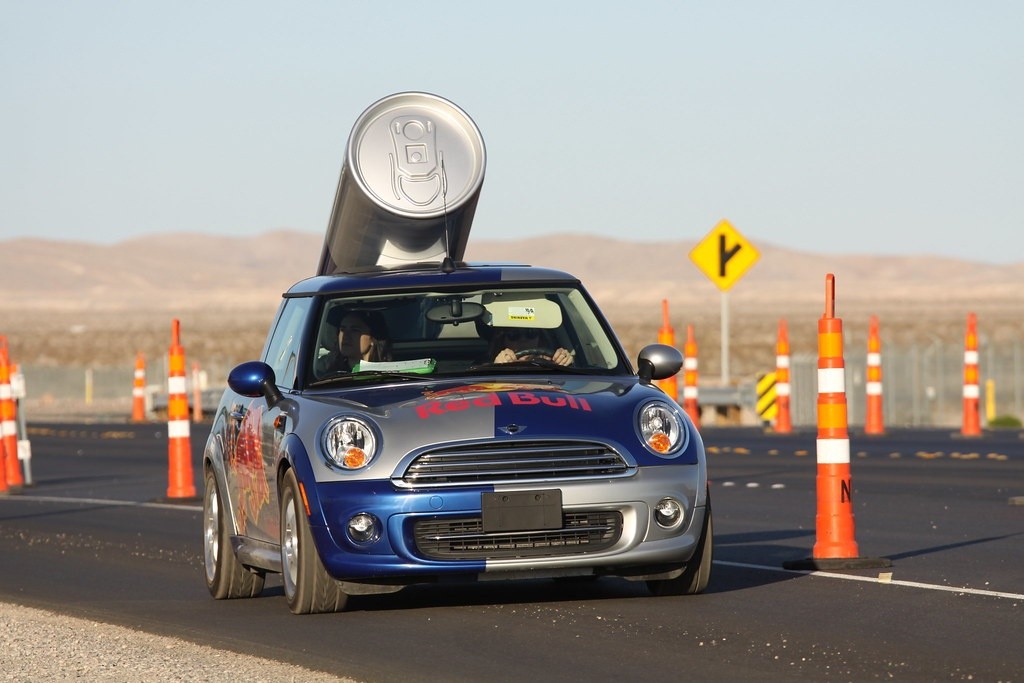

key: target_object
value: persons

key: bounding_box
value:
[319,309,392,375]
[493,327,575,367]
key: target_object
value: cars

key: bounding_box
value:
[202,260,711,616]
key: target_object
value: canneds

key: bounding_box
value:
[317,90,487,352]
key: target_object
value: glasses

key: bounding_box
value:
[506,329,539,341]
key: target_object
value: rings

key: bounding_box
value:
[562,353,567,356]
[505,353,509,356]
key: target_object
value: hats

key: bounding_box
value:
[326,302,388,340]
